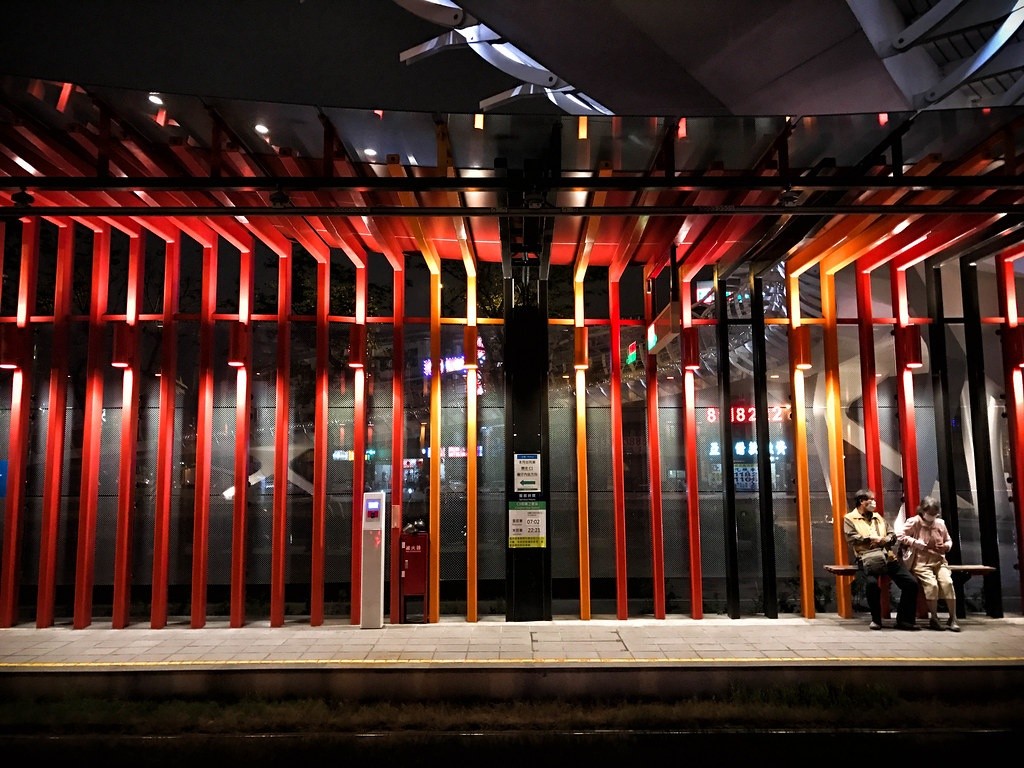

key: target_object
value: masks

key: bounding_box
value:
[923,512,940,522]
[863,499,876,512]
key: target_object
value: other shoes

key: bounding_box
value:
[945,618,961,632]
[893,620,921,630]
[868,621,882,629]
[928,618,946,631]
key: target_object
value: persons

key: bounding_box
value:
[843,489,921,630]
[896,495,960,632]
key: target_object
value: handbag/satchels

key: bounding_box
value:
[893,517,919,570]
[860,548,887,575]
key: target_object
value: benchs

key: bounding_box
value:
[823,564,995,619]
